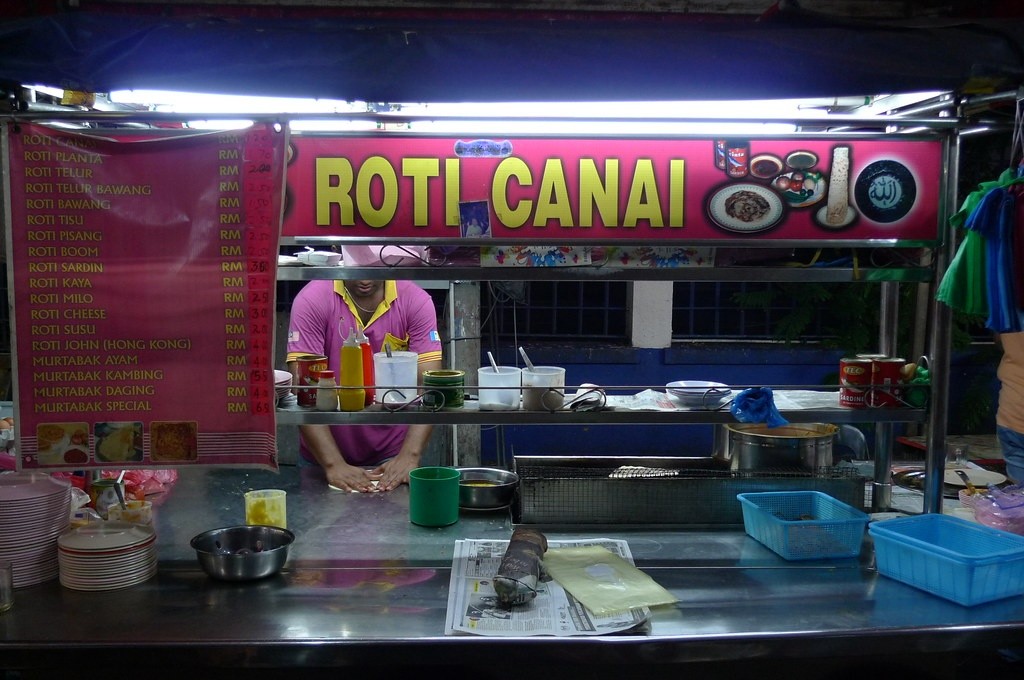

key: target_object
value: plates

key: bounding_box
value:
[0,472,157,589]
[785,150,819,170]
[771,171,828,208]
[274,369,294,402]
[703,180,786,234]
[749,153,783,179]
[666,380,729,405]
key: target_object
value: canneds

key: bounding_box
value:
[92,478,125,520]
[838,352,906,408]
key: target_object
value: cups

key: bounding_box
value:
[409,467,460,528]
[0,561,15,614]
[244,489,287,531]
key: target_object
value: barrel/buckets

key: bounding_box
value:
[107,501,154,528]
[91,479,125,521]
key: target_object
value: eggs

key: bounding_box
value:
[0,417,14,432]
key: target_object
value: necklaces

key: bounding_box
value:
[344,286,375,312]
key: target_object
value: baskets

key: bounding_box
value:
[736,491,869,561]
[869,512,1024,607]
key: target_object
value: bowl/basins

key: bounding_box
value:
[451,467,520,507]
[191,525,295,583]
[725,424,839,470]
[521,366,567,410]
[373,351,420,405]
[478,366,522,412]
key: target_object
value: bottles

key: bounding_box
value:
[338,328,366,412]
[316,370,338,411]
[714,139,750,179]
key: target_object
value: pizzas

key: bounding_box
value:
[37,424,66,443]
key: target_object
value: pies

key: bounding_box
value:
[98,425,134,462]
[154,422,191,458]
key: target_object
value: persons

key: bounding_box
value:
[995,331,1024,483]
[293,466,436,595]
[287,277,442,493]
[466,217,482,237]
